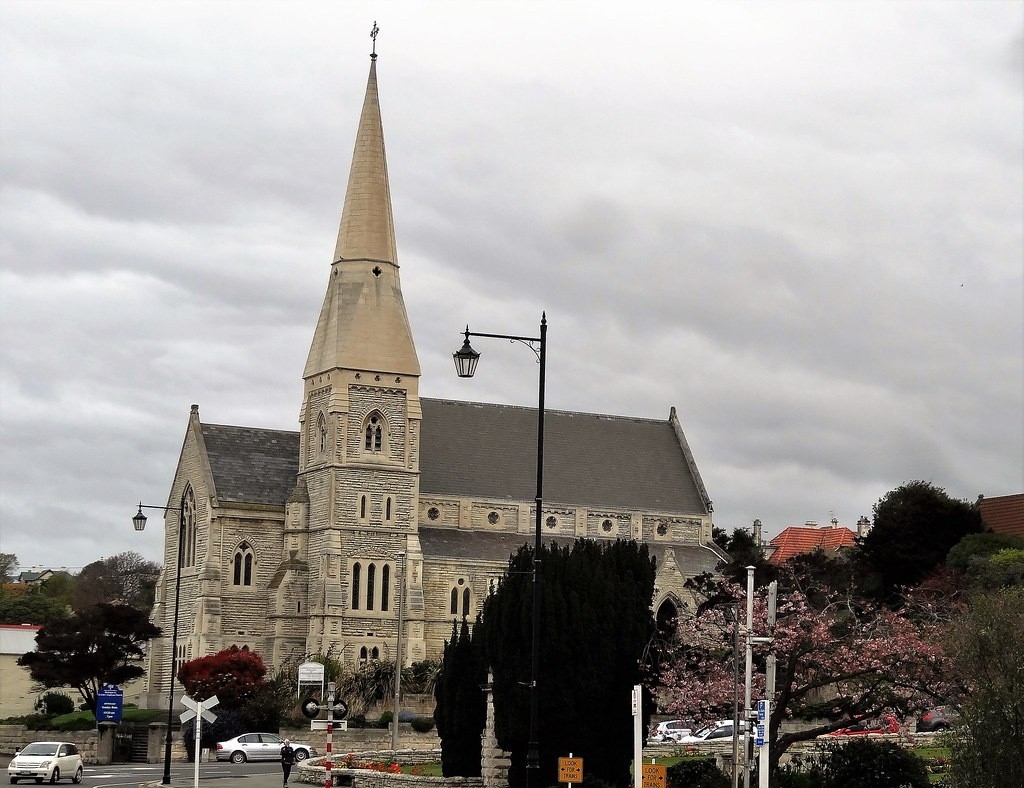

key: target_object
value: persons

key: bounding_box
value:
[280,738,295,788]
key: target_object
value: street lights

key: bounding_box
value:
[457,312,558,781]
[133,496,186,785]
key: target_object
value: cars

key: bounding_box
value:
[688,720,753,747]
[827,716,900,735]
[916,702,969,732]
[657,719,704,745]
[10,740,83,783]
[218,730,312,771]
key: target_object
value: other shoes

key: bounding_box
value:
[282,783,289,788]
[281,779,284,783]
